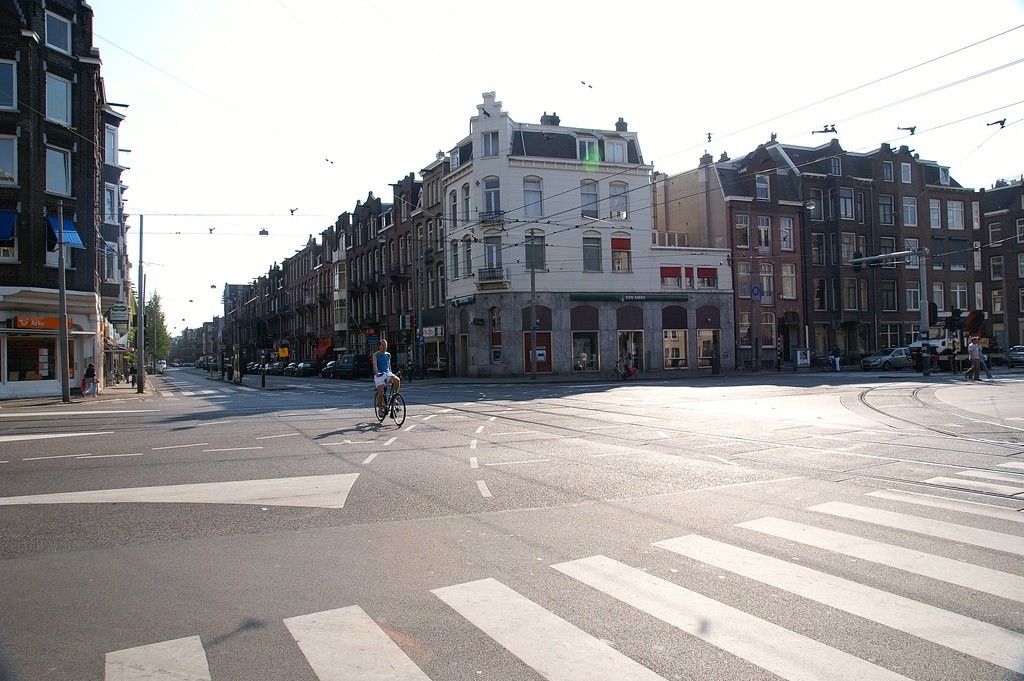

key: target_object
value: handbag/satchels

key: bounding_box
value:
[93,377,100,383]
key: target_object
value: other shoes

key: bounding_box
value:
[963,372,967,378]
[974,378,982,381]
[969,376,973,380]
[378,411,385,417]
[988,376,994,379]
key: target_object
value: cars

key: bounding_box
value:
[195,354,373,381]
[860,345,914,372]
[156,359,180,373]
[1004,345,1024,368]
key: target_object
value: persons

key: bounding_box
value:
[580,353,587,370]
[123,362,131,383]
[620,352,632,377]
[130,365,136,388]
[372,339,402,418]
[82,363,98,399]
[573,342,577,366]
[963,336,994,380]
[591,353,597,369]
[800,352,807,364]
[828,344,841,371]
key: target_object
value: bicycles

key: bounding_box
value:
[606,359,637,382]
[822,356,844,373]
[372,371,407,427]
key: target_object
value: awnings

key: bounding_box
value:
[103,337,129,353]
[313,345,332,354]
